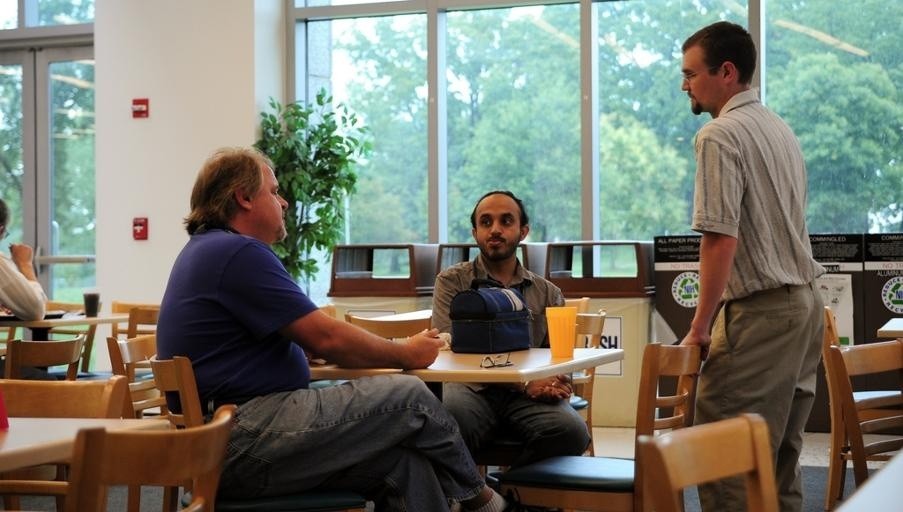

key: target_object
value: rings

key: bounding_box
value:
[550,381,557,387]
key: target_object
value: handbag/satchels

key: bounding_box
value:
[449,278,532,354]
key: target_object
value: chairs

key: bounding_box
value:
[636,410,780,511]
[822,306,902,512]
[563,296,590,400]
[568,308,607,457]
[344,313,432,341]
[499,343,702,511]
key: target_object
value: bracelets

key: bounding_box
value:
[524,380,531,394]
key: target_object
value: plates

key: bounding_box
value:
[0,310,66,320]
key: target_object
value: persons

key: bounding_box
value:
[157,145,563,512]
[430,190,588,510]
[678,20,826,512]
[0,199,60,382]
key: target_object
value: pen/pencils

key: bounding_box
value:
[527,309,534,320]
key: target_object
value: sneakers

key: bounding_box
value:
[501,493,564,511]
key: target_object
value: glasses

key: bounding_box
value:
[2,224,11,242]
[479,352,514,369]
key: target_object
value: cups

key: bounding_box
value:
[82,288,100,317]
[546,307,578,363]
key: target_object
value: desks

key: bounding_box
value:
[308,347,624,403]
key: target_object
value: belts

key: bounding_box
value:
[173,398,250,431]
